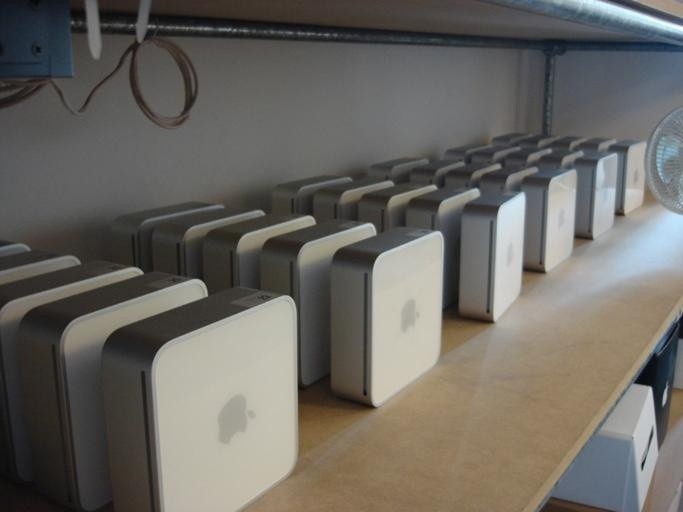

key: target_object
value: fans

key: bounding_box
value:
[644,106,683,215]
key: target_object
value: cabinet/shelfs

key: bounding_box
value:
[0,0,682,512]
